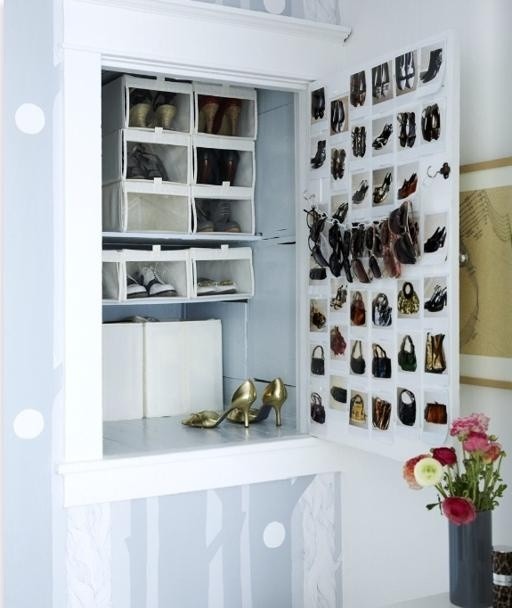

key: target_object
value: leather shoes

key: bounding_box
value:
[311,48,443,133]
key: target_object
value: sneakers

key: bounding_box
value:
[197,278,237,295]
[127,273,147,298]
[136,265,176,297]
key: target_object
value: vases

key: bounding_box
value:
[449,509,493,607]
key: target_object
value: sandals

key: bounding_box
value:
[129,89,153,127]
[331,148,346,181]
[351,103,440,158]
[153,91,178,130]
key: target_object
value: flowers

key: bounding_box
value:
[402,412,508,526]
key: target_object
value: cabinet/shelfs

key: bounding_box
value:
[55,0,462,506]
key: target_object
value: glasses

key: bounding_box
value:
[303,199,418,284]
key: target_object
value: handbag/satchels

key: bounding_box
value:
[123,144,169,181]
[350,291,366,325]
[311,300,326,329]
[310,267,327,280]
[372,293,392,326]
[397,282,419,314]
[309,326,447,430]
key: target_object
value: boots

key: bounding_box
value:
[311,140,327,170]
[192,199,214,232]
[198,149,219,183]
[210,200,240,232]
[217,150,240,185]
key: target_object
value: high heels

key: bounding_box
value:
[332,172,418,225]
[424,226,446,252]
[213,99,241,136]
[226,377,288,427]
[182,379,257,428]
[199,96,221,134]
[334,285,348,310]
[424,285,441,308]
[428,287,448,312]
[330,285,344,307]
